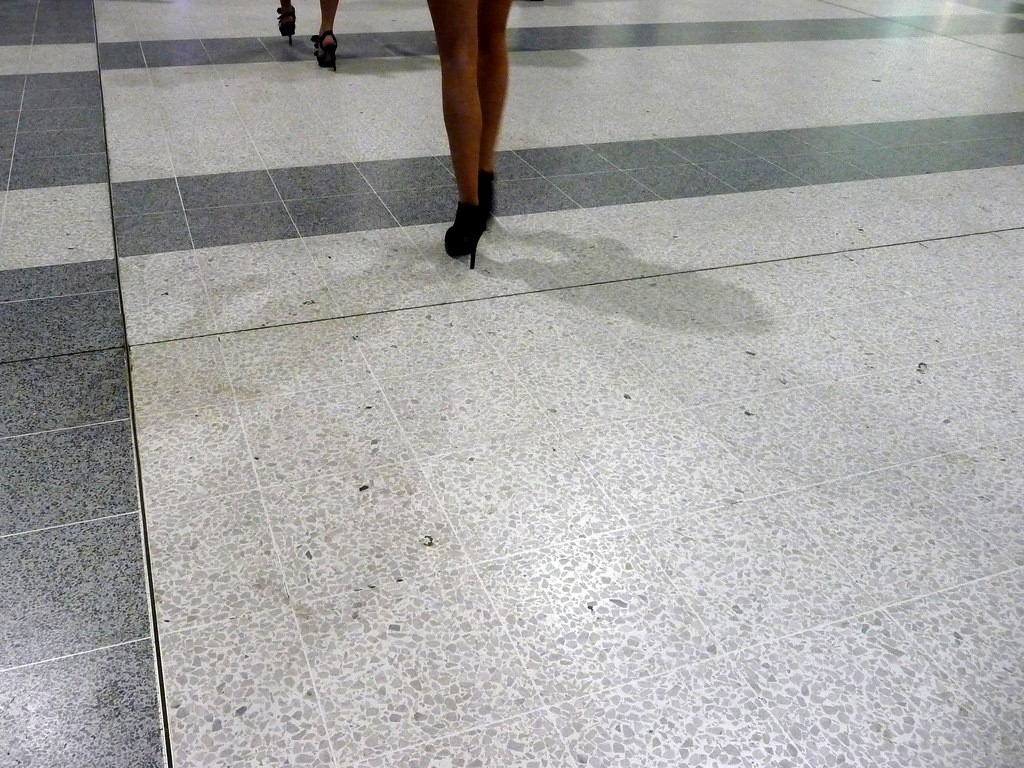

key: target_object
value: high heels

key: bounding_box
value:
[277,6,296,47]
[310,31,338,71]
[445,201,486,269]
[478,171,497,222]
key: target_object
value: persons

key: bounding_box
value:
[426,0,513,269]
[277,0,339,71]
